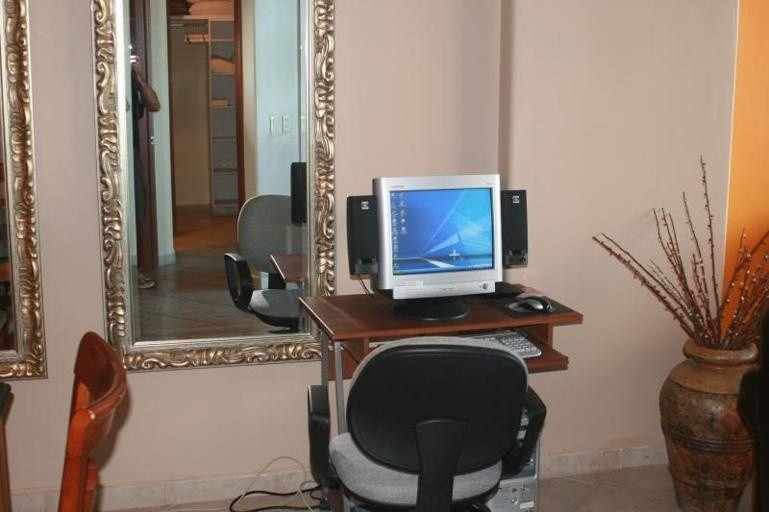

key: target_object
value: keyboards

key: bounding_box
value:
[377,330,541,359]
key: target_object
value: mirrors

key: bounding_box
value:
[0,0,49,381]
[88,0,338,374]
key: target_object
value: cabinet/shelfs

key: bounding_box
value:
[208,19,242,215]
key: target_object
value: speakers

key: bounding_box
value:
[501,190,528,269]
[347,196,379,274]
[291,162,306,225]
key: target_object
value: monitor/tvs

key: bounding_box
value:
[373,173,504,322]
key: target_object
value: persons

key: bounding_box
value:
[127,42,159,294]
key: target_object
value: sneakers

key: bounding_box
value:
[137,270,156,289]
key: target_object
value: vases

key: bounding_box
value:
[659,337,762,511]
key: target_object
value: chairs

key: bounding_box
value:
[56,330,128,510]
[305,335,548,510]
[222,195,304,333]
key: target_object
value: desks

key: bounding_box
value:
[295,283,583,507]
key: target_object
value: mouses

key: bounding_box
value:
[519,296,550,312]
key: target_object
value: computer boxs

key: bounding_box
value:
[483,407,540,512]
